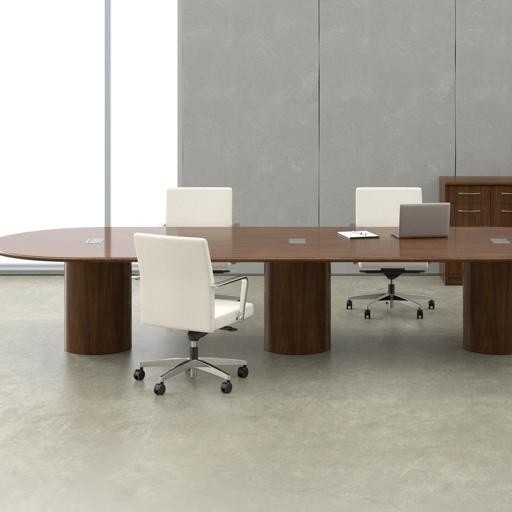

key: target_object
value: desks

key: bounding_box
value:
[0,226,512,355]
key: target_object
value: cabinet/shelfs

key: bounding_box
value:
[438,177,512,286]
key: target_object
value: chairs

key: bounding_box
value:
[132,233,253,396]
[346,188,435,320]
[164,186,233,335]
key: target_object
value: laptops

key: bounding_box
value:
[390,201,451,240]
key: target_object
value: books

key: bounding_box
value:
[336,230,378,239]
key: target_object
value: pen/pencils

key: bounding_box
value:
[360,231,366,236]
